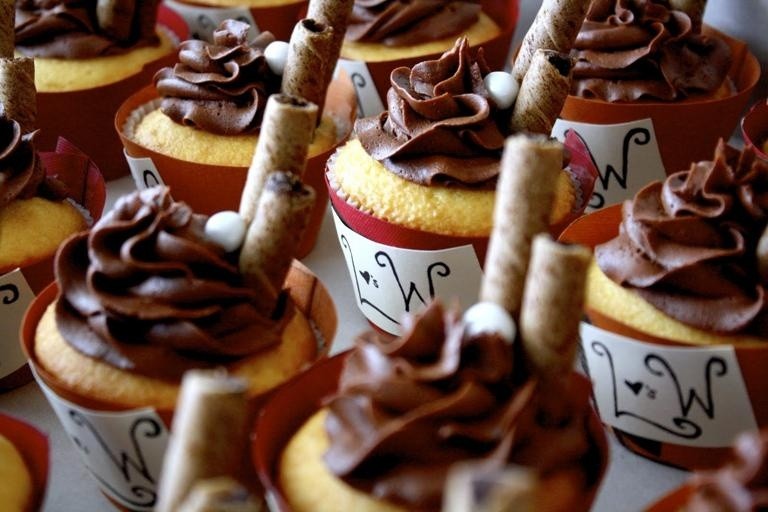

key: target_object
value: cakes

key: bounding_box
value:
[0,0,768,512]
